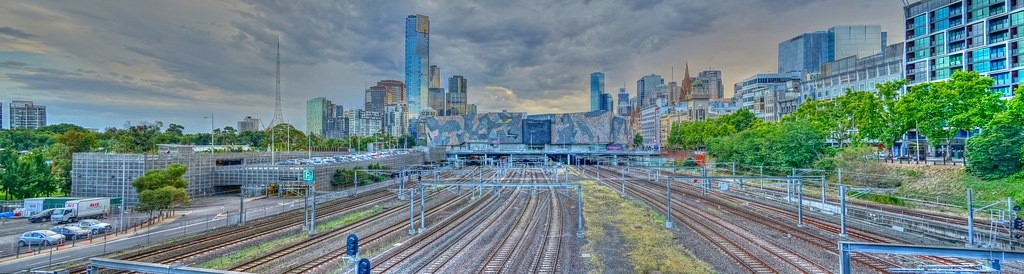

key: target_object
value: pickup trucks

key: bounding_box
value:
[66,219,112,234]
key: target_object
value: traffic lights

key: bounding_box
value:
[359,259,370,274]
[347,235,358,256]
[1014,218,1024,230]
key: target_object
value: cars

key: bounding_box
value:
[897,154,923,162]
[50,225,90,241]
[274,151,413,165]
[18,230,65,247]
[28,209,61,222]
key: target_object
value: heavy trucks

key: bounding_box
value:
[50,196,110,224]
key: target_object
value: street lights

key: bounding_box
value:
[203,114,214,155]
